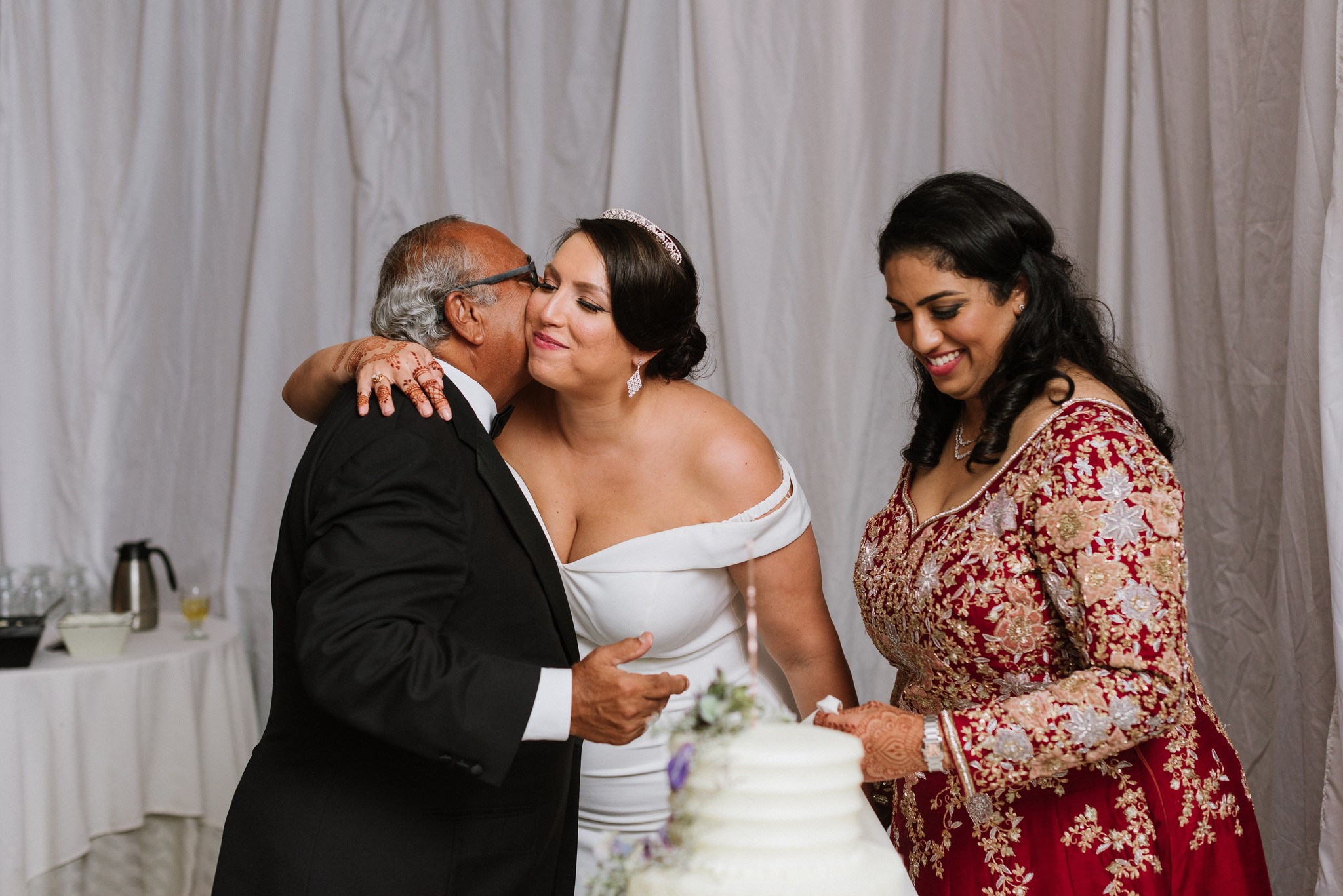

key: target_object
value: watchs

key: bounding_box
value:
[919,710,949,774]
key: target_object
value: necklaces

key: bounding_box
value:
[951,422,979,459]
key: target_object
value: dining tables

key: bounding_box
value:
[2,610,261,896]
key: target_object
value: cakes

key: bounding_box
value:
[596,720,916,896]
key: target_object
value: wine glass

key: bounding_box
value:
[178,583,211,642]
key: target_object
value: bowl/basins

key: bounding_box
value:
[58,607,133,662]
[1,613,45,668]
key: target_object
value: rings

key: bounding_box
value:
[369,372,393,389]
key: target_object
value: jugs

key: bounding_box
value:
[110,539,178,632]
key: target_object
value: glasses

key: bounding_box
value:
[428,253,544,322]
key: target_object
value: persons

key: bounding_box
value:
[811,171,1270,896]
[280,207,870,896]
[208,211,688,896]
